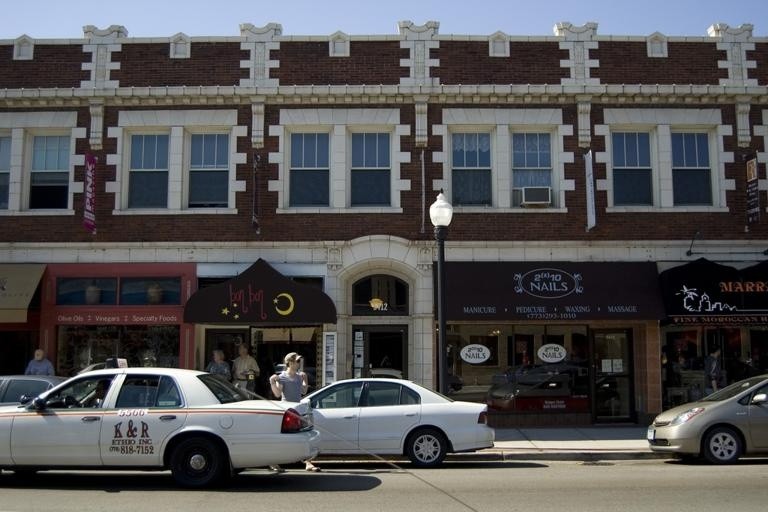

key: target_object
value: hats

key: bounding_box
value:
[285,352,302,363]
[35,349,43,360]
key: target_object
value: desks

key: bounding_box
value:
[680,371,705,400]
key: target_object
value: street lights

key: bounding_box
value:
[428,188,453,396]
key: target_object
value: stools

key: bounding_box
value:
[667,387,689,408]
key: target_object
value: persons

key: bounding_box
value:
[232,343,259,392]
[724,350,768,385]
[703,345,723,398]
[23,349,55,376]
[67,377,110,409]
[267,351,322,473]
[205,348,232,385]
[521,349,531,366]
[380,355,393,378]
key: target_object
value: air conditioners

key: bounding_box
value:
[520,186,552,205]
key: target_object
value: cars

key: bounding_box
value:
[0,357,320,489]
[76,362,106,374]
[369,369,402,379]
[646,374,767,465]
[486,365,620,414]
[274,364,286,374]
[0,376,61,408]
[298,378,494,468]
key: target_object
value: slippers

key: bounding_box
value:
[270,466,285,472]
[306,467,320,472]
